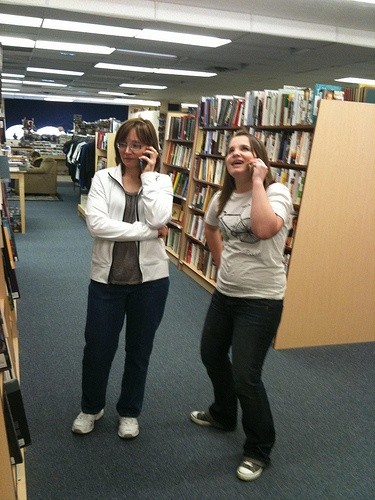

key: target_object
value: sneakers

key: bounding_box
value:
[118,413,139,439]
[235,455,263,481]
[72,409,104,433]
[191,411,236,431]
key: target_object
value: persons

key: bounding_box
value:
[189,129,294,481]
[71,119,174,438]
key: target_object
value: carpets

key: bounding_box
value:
[7,193,64,202]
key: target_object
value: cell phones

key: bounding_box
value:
[140,153,150,169]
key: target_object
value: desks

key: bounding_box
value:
[7,170,27,234]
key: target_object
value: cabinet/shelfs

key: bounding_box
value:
[76,97,375,350]
[0,250,27,500]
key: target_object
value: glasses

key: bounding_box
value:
[115,142,147,151]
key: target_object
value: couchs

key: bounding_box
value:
[14,156,58,195]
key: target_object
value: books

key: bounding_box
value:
[160,83,375,282]
